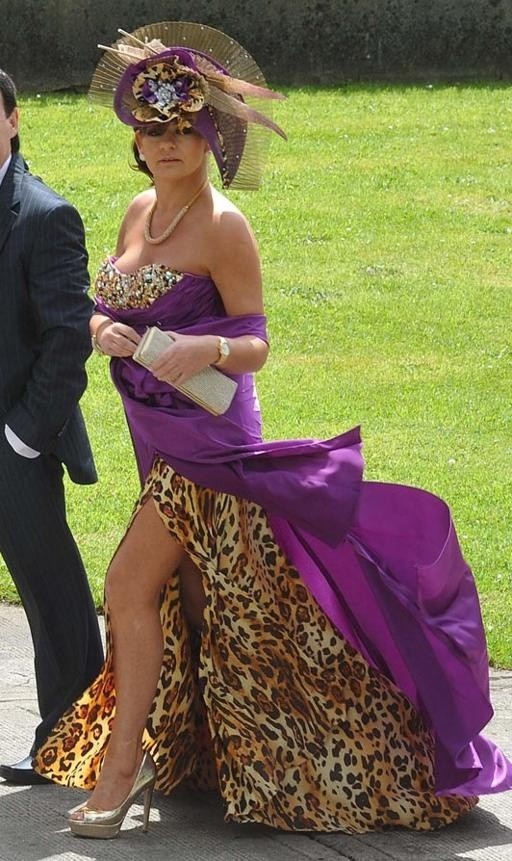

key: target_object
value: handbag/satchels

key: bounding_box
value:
[132,325,238,417]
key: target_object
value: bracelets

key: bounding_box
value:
[87,316,113,357]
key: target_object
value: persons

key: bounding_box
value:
[28,112,512,842]
[0,66,108,788]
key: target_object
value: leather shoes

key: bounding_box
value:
[0,754,58,786]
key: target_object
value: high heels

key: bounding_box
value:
[65,748,160,841]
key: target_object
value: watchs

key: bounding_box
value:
[214,329,230,371]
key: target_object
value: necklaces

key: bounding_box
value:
[142,179,209,247]
